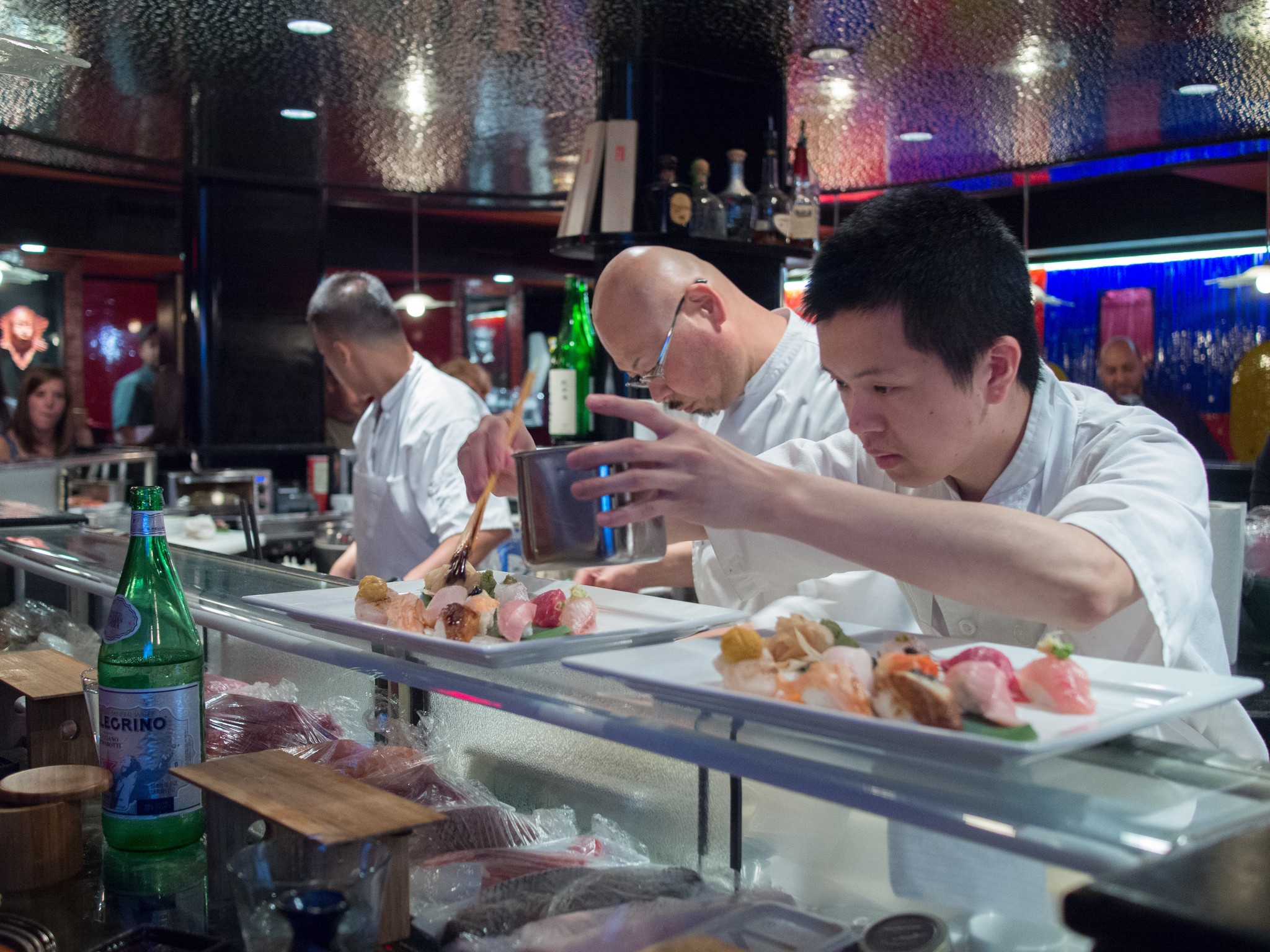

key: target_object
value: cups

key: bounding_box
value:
[80,668,101,770]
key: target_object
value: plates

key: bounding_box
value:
[1209,496,1248,666]
[238,568,750,670]
[558,619,1264,760]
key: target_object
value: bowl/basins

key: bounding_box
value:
[512,441,665,567]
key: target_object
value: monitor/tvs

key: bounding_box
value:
[1024,232,1269,472]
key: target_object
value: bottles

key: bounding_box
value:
[544,275,597,441]
[653,115,818,247]
[95,484,209,852]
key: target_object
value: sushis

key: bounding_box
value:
[339,549,1108,764]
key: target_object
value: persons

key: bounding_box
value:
[305,272,518,592]
[9,307,36,346]
[1091,335,1234,465]
[573,242,906,620]
[111,324,191,510]
[1,367,103,634]
[456,181,1270,773]
[433,356,489,402]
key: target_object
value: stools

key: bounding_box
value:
[3,645,101,774]
[167,743,439,942]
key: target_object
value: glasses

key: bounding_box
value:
[623,278,710,389]
